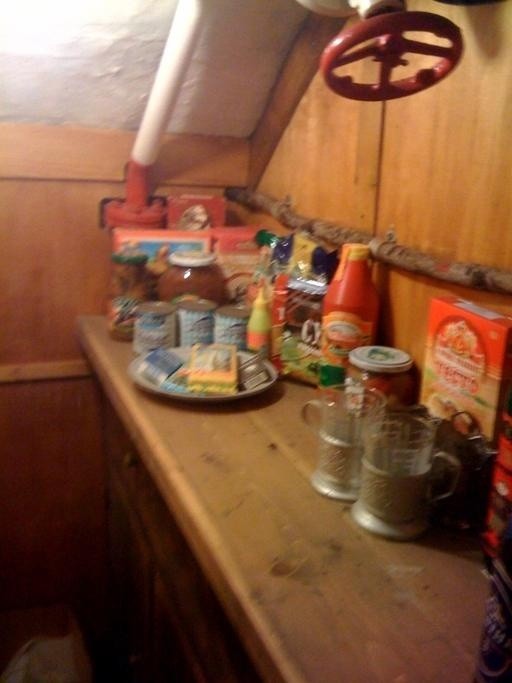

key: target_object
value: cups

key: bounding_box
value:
[299,378,462,544]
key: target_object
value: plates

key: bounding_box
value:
[126,347,280,403]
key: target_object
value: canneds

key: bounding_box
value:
[157,251,230,306]
[106,253,151,342]
[344,346,416,418]
[176,297,218,348]
[132,300,177,356]
[212,304,249,349]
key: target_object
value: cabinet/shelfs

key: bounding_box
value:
[98,378,264,682]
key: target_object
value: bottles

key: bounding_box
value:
[315,241,385,406]
[245,282,273,363]
